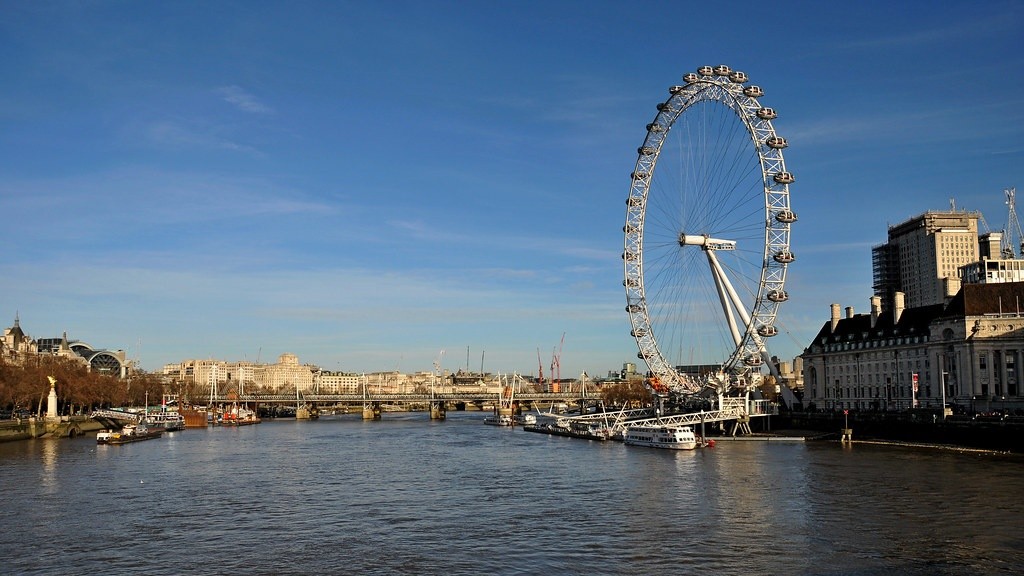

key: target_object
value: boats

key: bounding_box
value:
[625,423,695,450]
[91,409,185,432]
[96,424,165,446]
[484,414,511,426]
[524,414,607,441]
